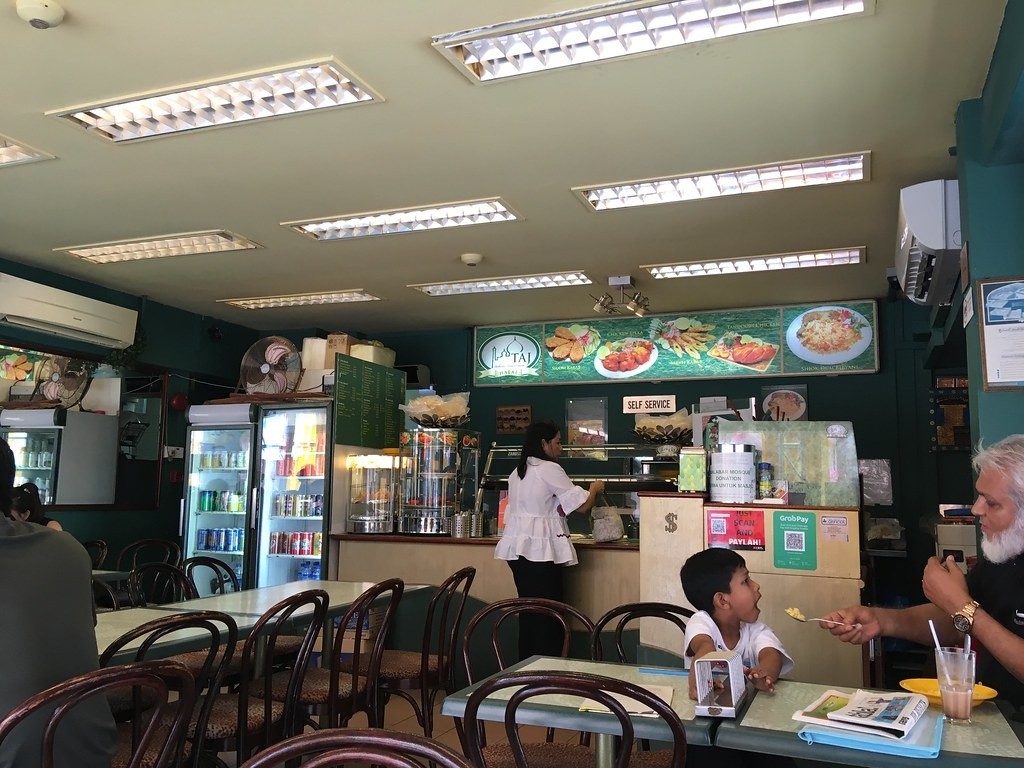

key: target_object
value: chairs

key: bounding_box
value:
[248,578,404,768]
[164,590,330,768]
[82,539,107,571]
[94,577,169,703]
[462,598,602,747]
[129,561,241,674]
[591,602,696,749]
[0,660,195,768]
[339,566,476,767]
[464,671,688,767]
[99,611,237,767]
[115,539,180,603]
[180,556,305,654]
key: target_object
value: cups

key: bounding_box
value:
[935,647,976,725]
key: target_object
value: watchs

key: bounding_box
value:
[951,600,979,634]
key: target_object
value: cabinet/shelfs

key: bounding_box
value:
[475,444,685,539]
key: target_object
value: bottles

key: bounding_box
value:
[297,561,321,582]
[18,477,53,505]
[758,463,772,499]
[230,564,242,593]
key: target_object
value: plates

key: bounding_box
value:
[548,326,601,363]
[786,305,872,365]
[594,338,658,379]
[762,390,806,421]
[707,332,781,373]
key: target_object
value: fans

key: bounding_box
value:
[35,353,92,407]
[239,335,301,396]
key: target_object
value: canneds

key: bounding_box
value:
[200,490,246,512]
[277,424,326,477]
[271,531,322,556]
[15,439,53,504]
[198,527,244,552]
[276,494,323,516]
[204,450,246,468]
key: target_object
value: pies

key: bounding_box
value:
[668,324,716,359]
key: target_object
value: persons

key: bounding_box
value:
[820,435,1024,747]
[0,438,118,768]
[494,423,605,662]
[680,548,796,700]
[11,483,63,532]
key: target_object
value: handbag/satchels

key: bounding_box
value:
[590,488,624,542]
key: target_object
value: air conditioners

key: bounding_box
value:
[894,180,960,307]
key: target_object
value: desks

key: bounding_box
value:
[441,656,1024,768]
[95,580,430,730]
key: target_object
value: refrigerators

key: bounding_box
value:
[252,399,327,585]
[0,408,119,505]
[178,402,258,602]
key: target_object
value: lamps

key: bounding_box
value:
[592,275,648,319]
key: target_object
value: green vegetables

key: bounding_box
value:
[851,316,868,334]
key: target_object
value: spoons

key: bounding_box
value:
[784,609,858,627]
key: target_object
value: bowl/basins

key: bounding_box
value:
[899,678,998,708]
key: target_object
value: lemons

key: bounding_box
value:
[740,335,763,347]
[597,346,611,360]
[675,318,691,330]
